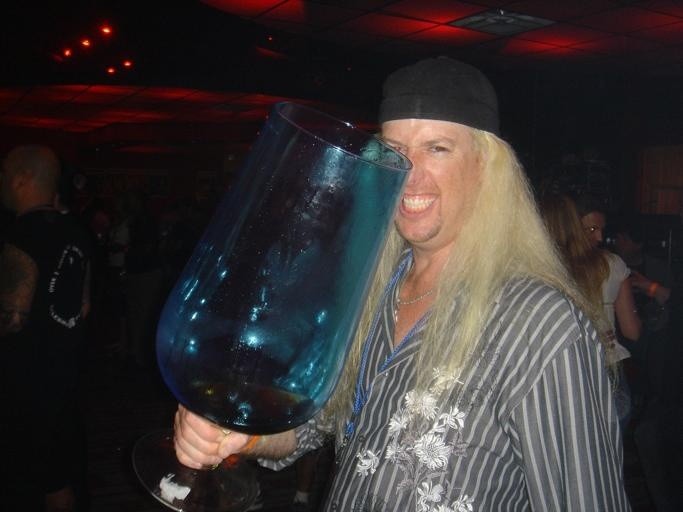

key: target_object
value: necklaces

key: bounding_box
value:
[392,262,443,323]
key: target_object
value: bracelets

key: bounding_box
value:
[238,432,264,458]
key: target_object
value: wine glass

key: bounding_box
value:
[130,100,414,512]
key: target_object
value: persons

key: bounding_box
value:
[2,143,320,512]
[168,54,635,511]
[548,192,671,429]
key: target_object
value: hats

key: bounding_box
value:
[378,55,500,138]
